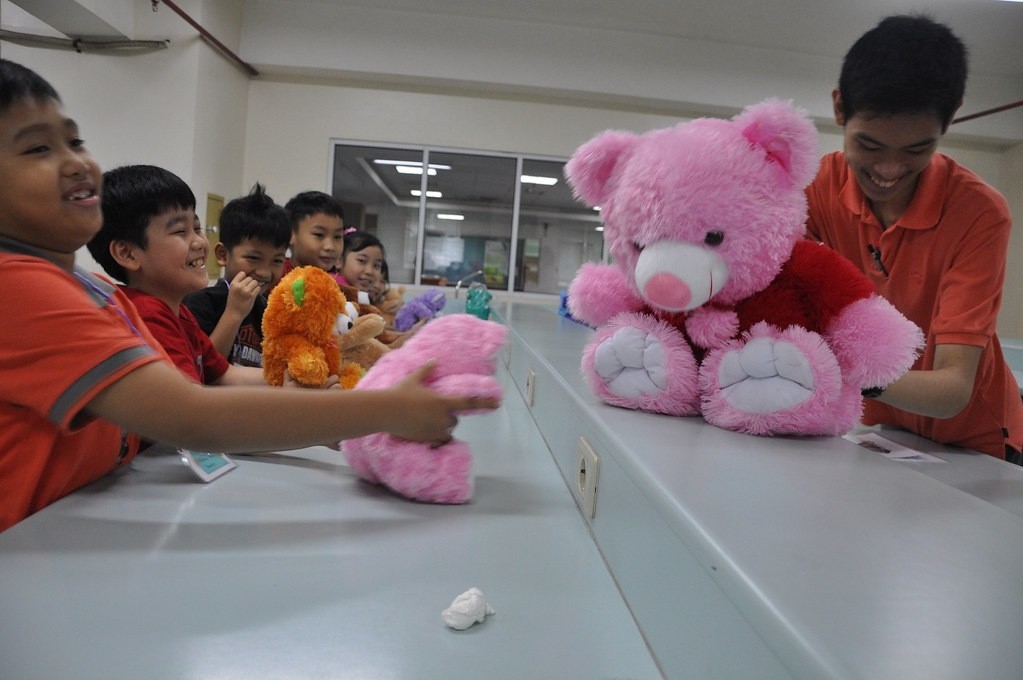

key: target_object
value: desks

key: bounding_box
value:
[844,416,1023,519]
[0,352,667,680]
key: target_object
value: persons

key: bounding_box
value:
[802,14,1023,467]
[0,59,498,533]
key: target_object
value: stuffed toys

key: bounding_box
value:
[564,96,924,436]
[261,265,510,503]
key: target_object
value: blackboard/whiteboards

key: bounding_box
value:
[420,234,526,290]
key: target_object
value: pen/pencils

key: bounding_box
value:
[867,243,888,277]
